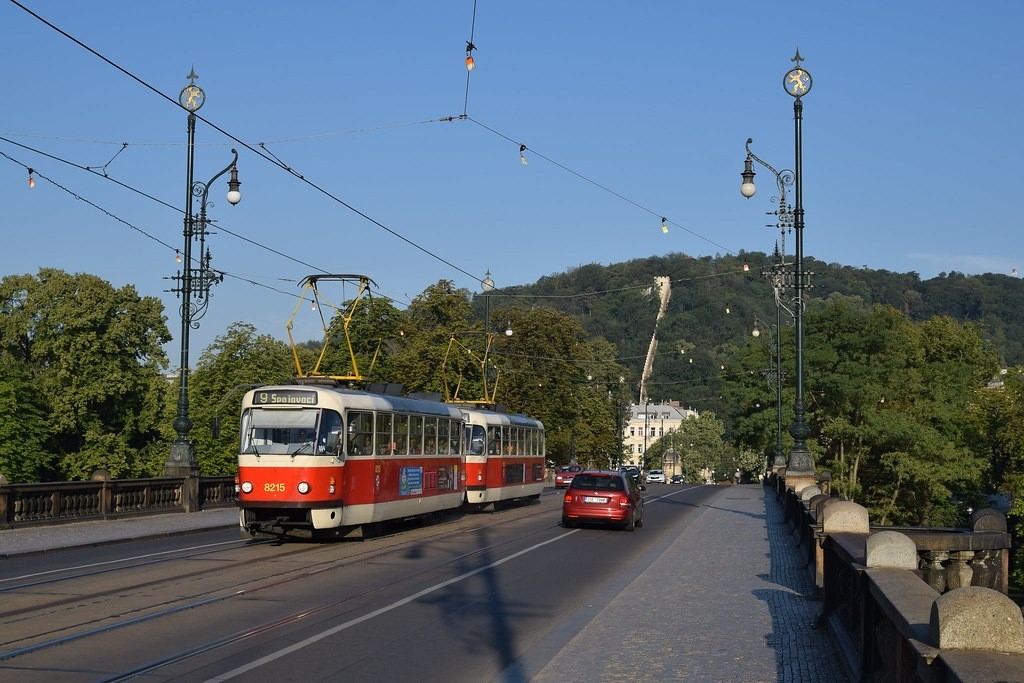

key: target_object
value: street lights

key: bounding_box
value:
[160,61,243,476]
[469,266,514,398]
[752,240,788,471]
[740,47,815,473]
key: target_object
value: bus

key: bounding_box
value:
[231,273,547,547]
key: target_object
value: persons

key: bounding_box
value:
[361,436,380,455]
[297,429,315,446]
[544,466,550,485]
[488,442,536,456]
[384,436,475,455]
[333,431,358,456]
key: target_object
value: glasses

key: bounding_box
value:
[297,432,306,435]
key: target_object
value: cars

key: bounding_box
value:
[618,465,644,485]
[669,475,685,485]
[561,467,647,533]
[645,469,667,485]
[554,463,583,489]
